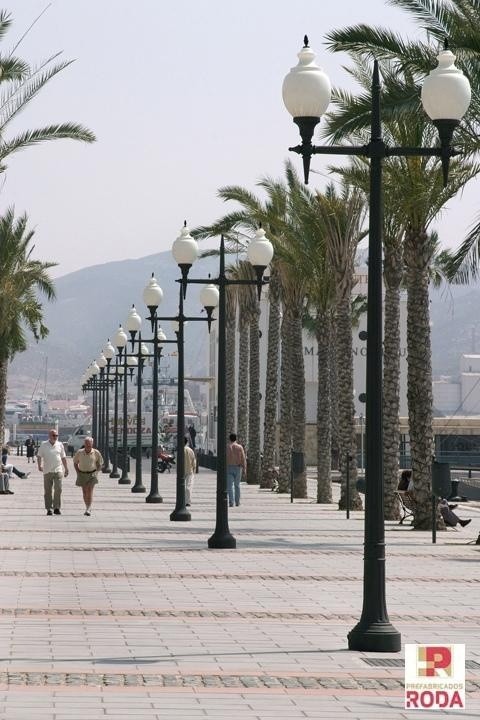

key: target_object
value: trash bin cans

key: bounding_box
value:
[292,452,305,473]
[68,444,74,452]
[432,462,453,498]
[0,473,10,494]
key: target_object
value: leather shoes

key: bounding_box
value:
[47,509,61,515]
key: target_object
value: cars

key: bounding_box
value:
[7,439,45,448]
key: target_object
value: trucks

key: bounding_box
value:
[157,415,201,436]
[67,412,153,459]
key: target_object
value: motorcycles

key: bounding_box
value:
[157,444,176,473]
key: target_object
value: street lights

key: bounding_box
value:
[172,219,275,549]
[143,272,219,521]
[281,34,471,654]
[126,303,187,503]
[80,323,167,493]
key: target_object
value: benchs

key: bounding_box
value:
[393,491,447,531]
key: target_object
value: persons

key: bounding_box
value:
[0,429,104,516]
[398,470,472,528]
[184,436,196,506]
[225,433,247,507]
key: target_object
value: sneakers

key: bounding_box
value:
[461,519,471,527]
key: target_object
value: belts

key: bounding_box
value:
[80,470,96,474]
